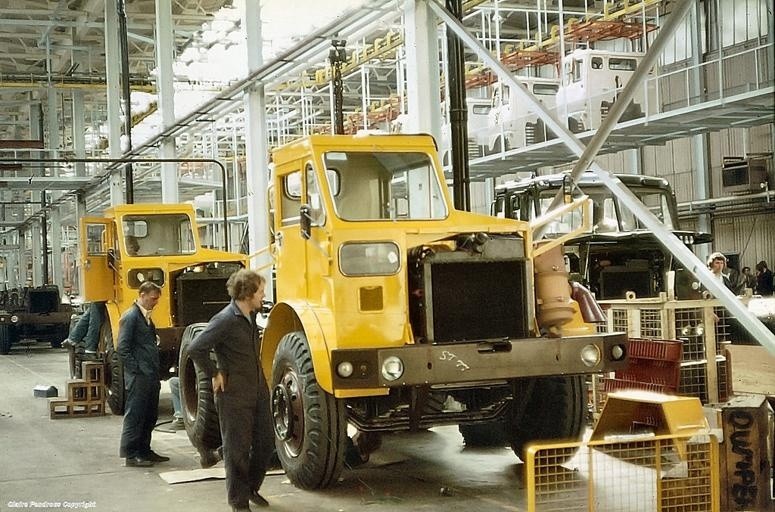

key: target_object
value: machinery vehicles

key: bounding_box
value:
[68,203,249,416]
[178,133,629,491]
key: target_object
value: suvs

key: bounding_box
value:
[0,285,76,354]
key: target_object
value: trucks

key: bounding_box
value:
[488,171,729,405]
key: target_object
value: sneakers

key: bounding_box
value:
[126,456,154,468]
[145,449,170,462]
[248,488,270,507]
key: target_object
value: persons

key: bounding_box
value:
[61,303,103,361]
[117,281,169,467]
[126,221,135,236]
[125,236,140,257]
[187,269,276,512]
[707,251,773,297]
